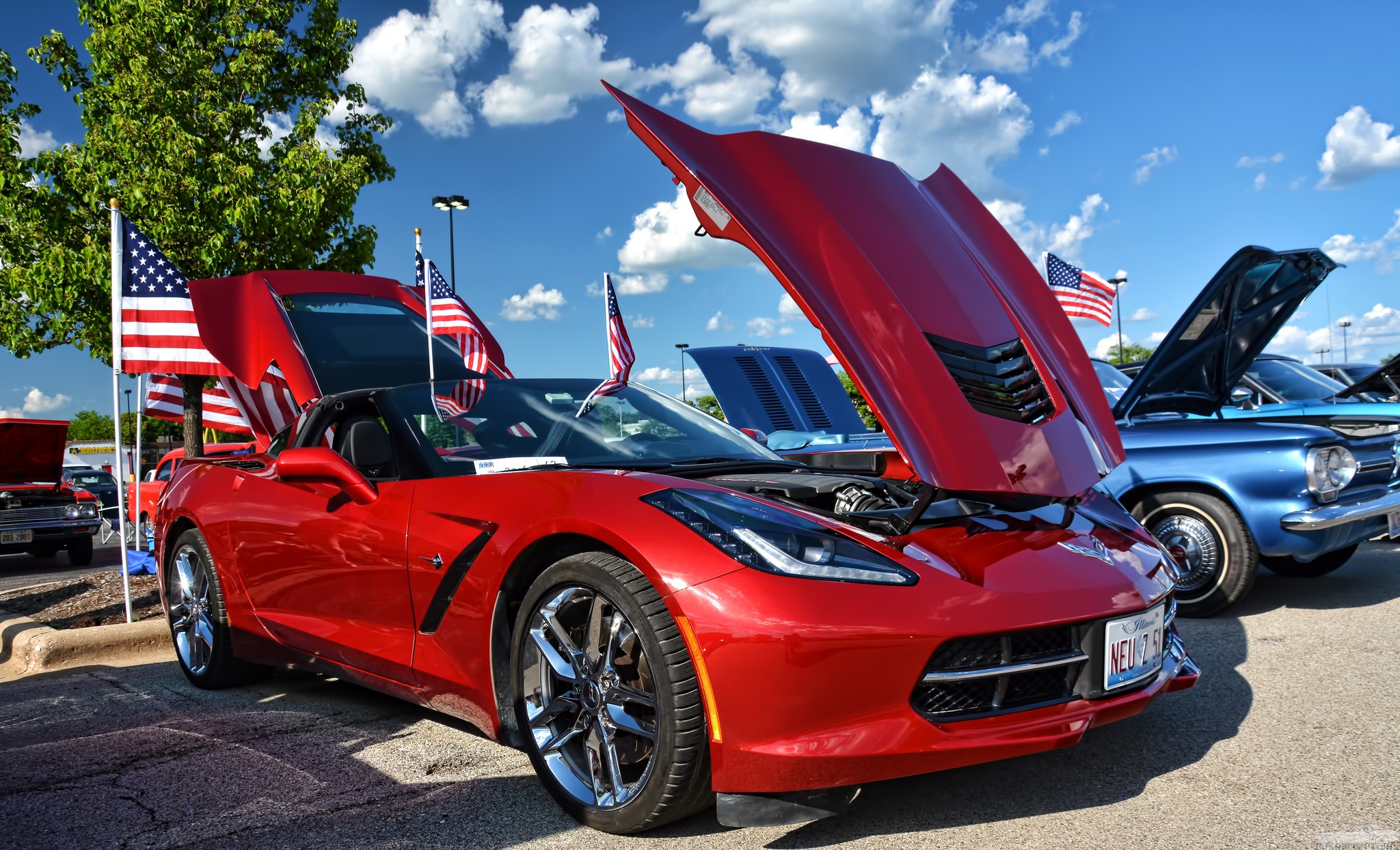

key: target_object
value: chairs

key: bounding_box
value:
[537,402,605,463]
[73,478,82,483]
[98,477,109,483]
[340,420,400,480]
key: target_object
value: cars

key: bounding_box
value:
[61,463,128,519]
[127,442,249,541]
[0,418,103,566]
[1112,353,1399,406]
[143,469,176,482]
[680,242,1399,621]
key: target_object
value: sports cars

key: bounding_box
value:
[151,78,1205,841]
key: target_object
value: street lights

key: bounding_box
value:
[615,401,625,438]
[1315,349,1330,364]
[675,344,689,401]
[432,195,470,295]
[1338,322,1351,363]
[124,390,136,483]
[1106,278,1127,366]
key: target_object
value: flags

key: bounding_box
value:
[576,379,627,418]
[432,379,537,439]
[413,249,515,379]
[1044,252,1116,328]
[143,373,255,437]
[603,273,635,381]
[110,209,336,450]
